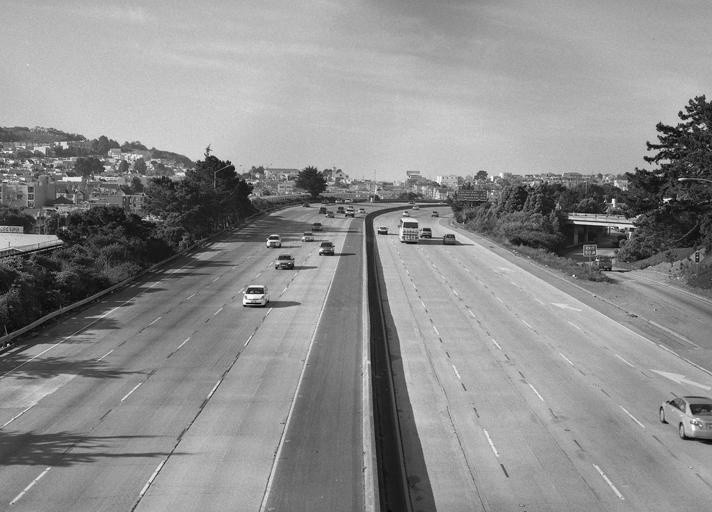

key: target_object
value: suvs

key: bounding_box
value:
[320,241,335,255]
[420,228,432,238]
[443,234,455,244]
[592,255,612,270]
[266,234,282,248]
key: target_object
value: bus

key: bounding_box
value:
[398,217,419,242]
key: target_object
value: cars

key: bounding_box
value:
[378,226,387,235]
[660,395,712,445]
[274,254,295,269]
[298,198,365,242]
[402,200,439,217]
[242,284,270,308]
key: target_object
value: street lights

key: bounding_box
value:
[212,164,232,190]
[678,177,712,185]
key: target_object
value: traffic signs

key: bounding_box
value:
[583,244,598,257]
